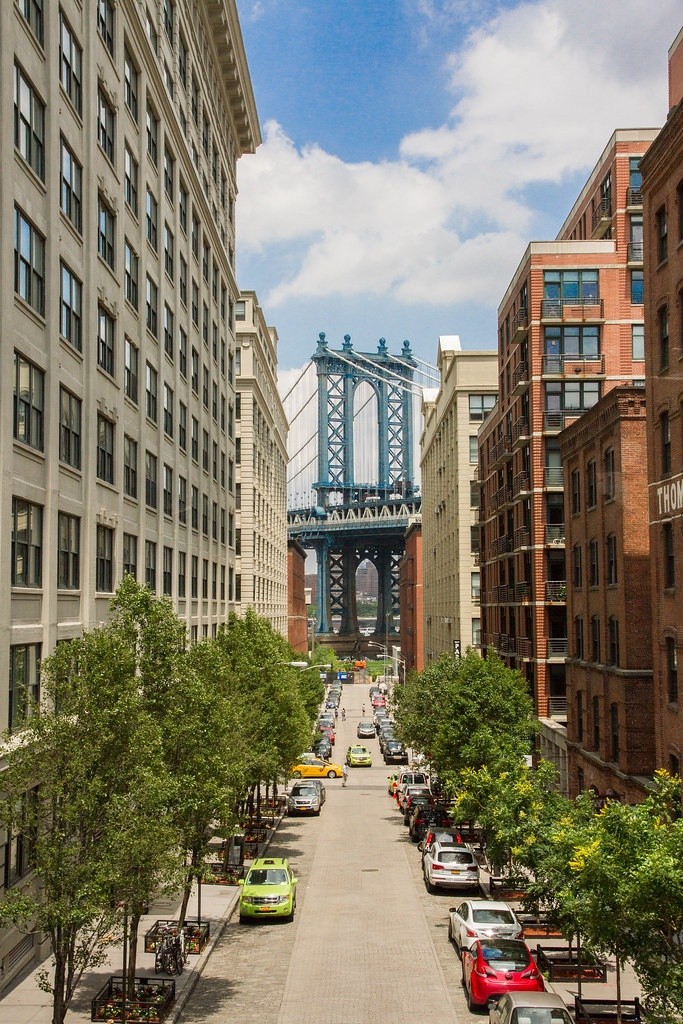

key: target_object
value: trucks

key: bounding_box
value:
[397,771,428,798]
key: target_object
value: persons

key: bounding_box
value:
[341,708,346,722]
[342,762,348,787]
[362,703,365,717]
[335,709,338,720]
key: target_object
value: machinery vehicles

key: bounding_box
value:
[350,639,366,670]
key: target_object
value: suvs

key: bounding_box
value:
[285,758,343,778]
[423,842,480,893]
[286,782,321,816]
[404,794,435,826]
[236,858,296,925]
[399,784,432,815]
[346,745,371,767]
[408,803,451,843]
[356,721,375,738]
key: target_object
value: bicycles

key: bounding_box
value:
[154,925,188,976]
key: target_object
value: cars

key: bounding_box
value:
[303,780,325,806]
[459,939,545,1012]
[447,901,525,960]
[311,679,343,763]
[369,687,407,763]
[488,991,575,1024]
[417,827,464,859]
[387,774,398,795]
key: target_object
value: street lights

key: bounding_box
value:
[376,655,405,688]
[367,645,389,676]
[368,640,385,684]
[244,661,307,675]
[299,664,331,672]
